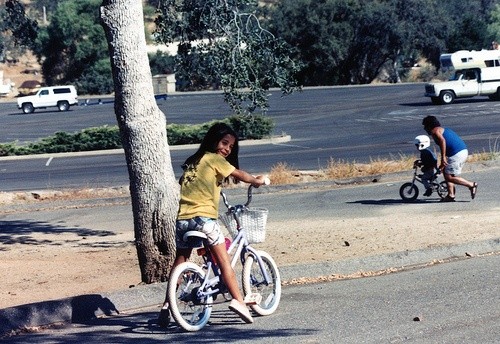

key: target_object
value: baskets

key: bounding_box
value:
[218,207,269,244]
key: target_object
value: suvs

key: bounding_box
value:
[17,85,78,113]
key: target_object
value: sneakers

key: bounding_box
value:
[158,306,171,330]
[228,298,254,324]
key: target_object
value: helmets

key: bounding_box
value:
[414,134,431,151]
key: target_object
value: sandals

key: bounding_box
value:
[469,182,478,199]
[440,195,456,202]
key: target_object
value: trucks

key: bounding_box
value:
[424,49,500,104]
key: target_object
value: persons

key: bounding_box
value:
[414,135,437,197]
[160,122,264,324]
[421,115,478,202]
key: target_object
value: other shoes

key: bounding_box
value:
[423,188,433,196]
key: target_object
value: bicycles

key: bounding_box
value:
[166,179,282,333]
[400,164,457,201]
